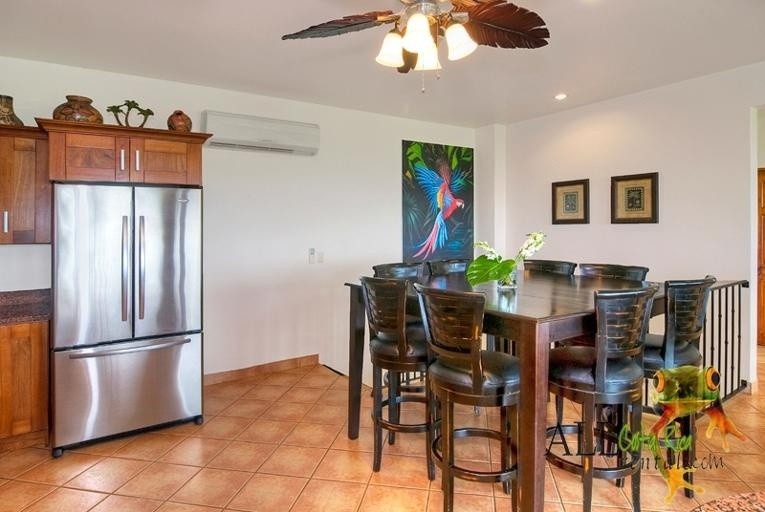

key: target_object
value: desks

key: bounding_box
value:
[344,270,666,512]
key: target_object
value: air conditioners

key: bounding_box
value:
[202,109,322,157]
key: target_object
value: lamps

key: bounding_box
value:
[282,0,552,73]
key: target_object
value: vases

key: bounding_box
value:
[497,264,520,293]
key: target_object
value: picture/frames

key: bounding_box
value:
[552,179,591,225]
[610,173,660,223]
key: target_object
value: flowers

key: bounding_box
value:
[464,230,547,287]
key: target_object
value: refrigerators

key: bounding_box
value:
[49,179,203,458]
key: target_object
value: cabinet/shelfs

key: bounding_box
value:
[1,124,53,244]
[35,117,213,186]
[1,320,52,451]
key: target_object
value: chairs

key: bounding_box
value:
[428,257,472,277]
[578,263,649,283]
[548,284,659,512]
[606,275,717,498]
[522,259,578,276]
[372,262,430,423]
[358,276,445,481]
[412,281,521,512]
